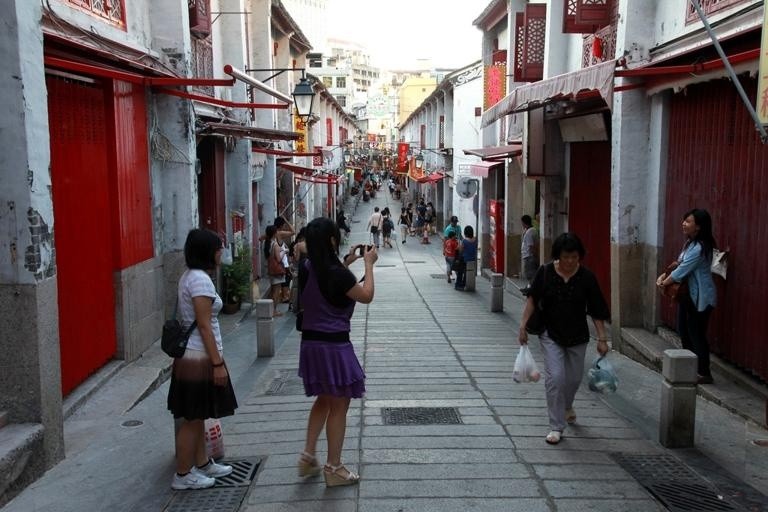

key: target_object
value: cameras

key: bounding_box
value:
[360,245,371,256]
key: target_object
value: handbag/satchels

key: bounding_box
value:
[161,319,187,358]
[371,226,377,233]
[660,260,688,300]
[525,298,545,335]
[268,256,286,276]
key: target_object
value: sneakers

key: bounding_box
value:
[171,458,232,489]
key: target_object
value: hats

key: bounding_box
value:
[450,216,458,222]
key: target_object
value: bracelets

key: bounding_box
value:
[212,361,224,367]
[598,340,606,342]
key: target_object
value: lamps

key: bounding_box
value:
[244,66,318,127]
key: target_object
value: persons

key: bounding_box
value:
[363,166,478,292]
[656,207,719,385]
[519,232,608,445]
[519,215,539,296]
[298,216,379,488]
[337,211,350,245]
[259,216,311,317]
[167,227,238,491]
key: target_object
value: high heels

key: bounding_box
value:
[298,451,360,487]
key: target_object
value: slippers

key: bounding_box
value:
[546,408,576,444]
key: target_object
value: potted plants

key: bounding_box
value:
[217,235,257,315]
